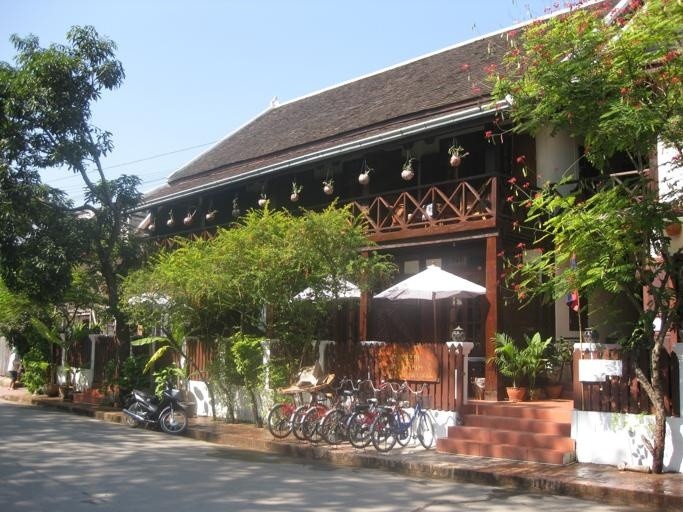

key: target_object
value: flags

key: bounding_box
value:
[561,250,580,312]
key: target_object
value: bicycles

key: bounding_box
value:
[266,376,434,452]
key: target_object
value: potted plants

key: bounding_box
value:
[448,146,469,167]
[322,179,335,194]
[183,210,197,224]
[401,158,415,181]
[166,209,174,226]
[205,210,216,220]
[358,168,373,184]
[19,346,128,409]
[291,183,302,201]
[258,193,267,206]
[483,330,573,402]
[148,217,156,231]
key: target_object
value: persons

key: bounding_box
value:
[6,346,17,390]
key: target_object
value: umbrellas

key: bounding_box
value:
[288,274,378,304]
[127,289,175,336]
[373,265,486,342]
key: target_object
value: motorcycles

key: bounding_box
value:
[123,374,188,436]
